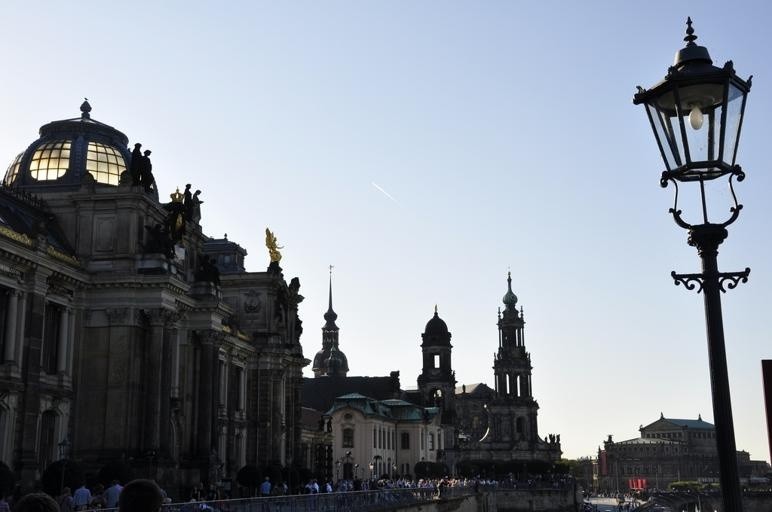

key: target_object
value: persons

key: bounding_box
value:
[192,190,204,224]
[130,143,143,185]
[140,150,154,193]
[183,184,194,219]
[266,228,284,262]
[1,454,666,512]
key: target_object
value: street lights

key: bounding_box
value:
[334,458,341,480]
[56,433,72,495]
[369,462,373,479]
[632,15,753,512]
